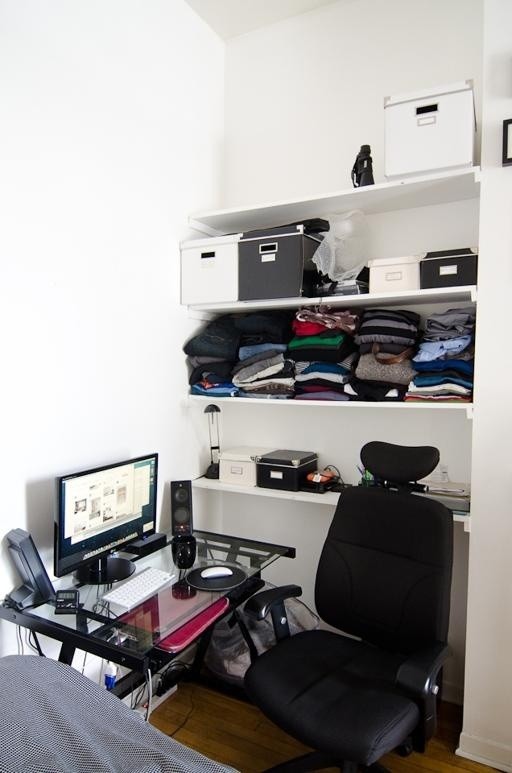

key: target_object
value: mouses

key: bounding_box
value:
[200,566,234,579]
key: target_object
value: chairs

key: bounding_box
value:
[241,439,456,771]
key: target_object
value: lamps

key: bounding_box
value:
[203,403,223,476]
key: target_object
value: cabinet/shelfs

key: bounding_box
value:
[182,166,481,532]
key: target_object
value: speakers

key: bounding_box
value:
[170,479,194,537]
[5,527,56,610]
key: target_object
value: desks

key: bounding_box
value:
[1,524,298,704]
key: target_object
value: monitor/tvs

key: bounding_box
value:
[54,452,159,585]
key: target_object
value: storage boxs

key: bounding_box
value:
[380,78,479,179]
[217,447,278,486]
[256,449,318,492]
[367,254,424,293]
[239,223,325,302]
[181,232,243,305]
[420,247,477,289]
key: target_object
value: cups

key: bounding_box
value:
[171,534,195,568]
[171,579,196,599]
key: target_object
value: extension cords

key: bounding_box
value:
[143,684,177,714]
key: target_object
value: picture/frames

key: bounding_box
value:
[503,118,512,166]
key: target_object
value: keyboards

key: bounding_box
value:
[101,565,175,611]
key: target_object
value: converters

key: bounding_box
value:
[164,669,177,691]
